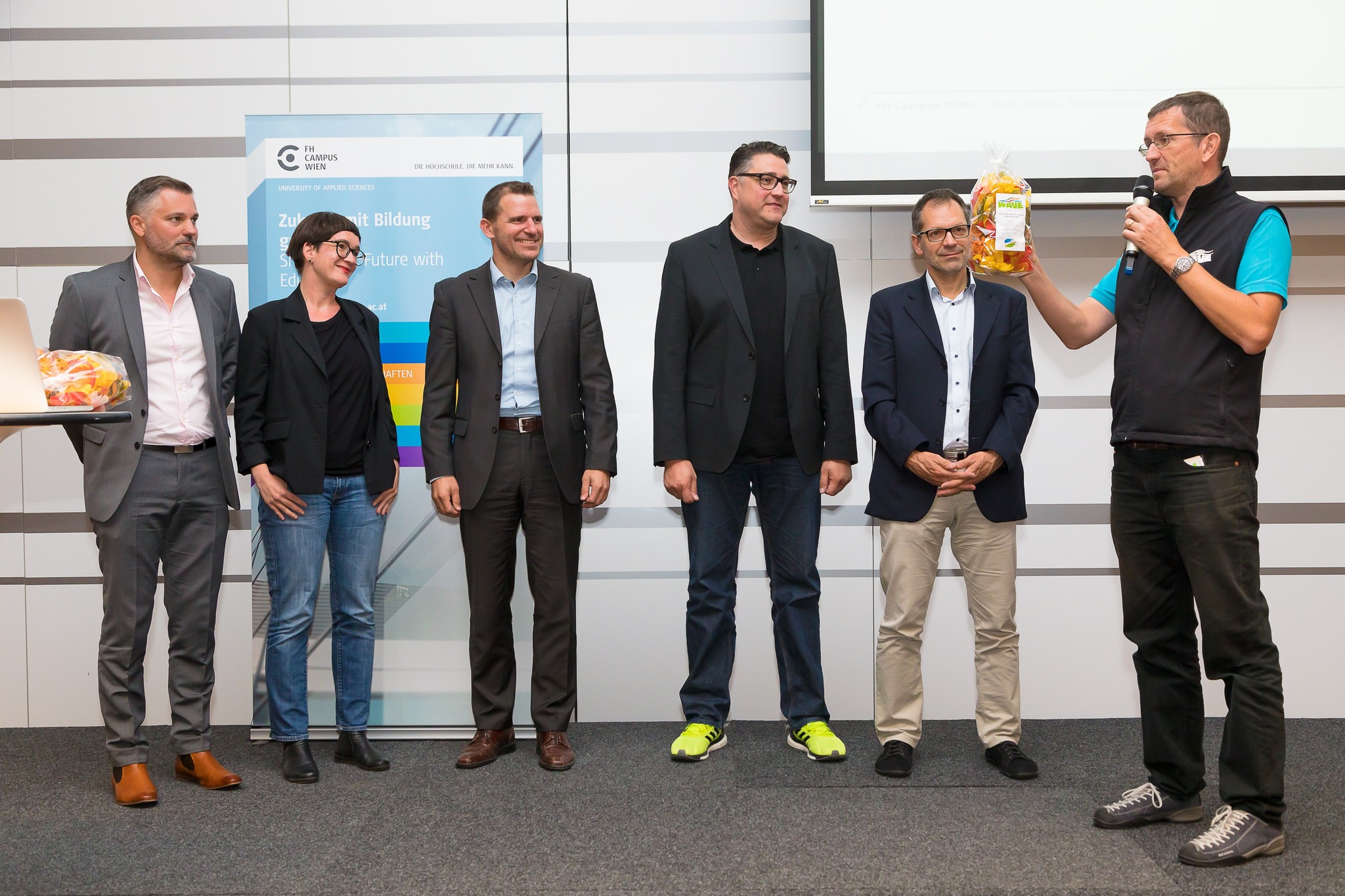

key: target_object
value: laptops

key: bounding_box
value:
[0,297,94,414]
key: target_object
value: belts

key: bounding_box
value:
[1113,441,1189,451]
[142,436,216,454]
[943,450,968,463]
[497,416,544,433]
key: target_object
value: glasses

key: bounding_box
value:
[1138,132,1209,157]
[916,224,971,242]
[316,240,366,266]
[737,173,797,193]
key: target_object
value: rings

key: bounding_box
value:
[1128,221,1136,230]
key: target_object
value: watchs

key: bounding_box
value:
[1170,256,1197,282]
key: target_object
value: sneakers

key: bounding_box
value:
[1092,783,1203,828]
[874,739,913,777]
[787,721,846,762]
[670,723,727,760]
[984,740,1039,779]
[1177,805,1286,865]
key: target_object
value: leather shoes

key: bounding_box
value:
[111,763,157,805]
[535,731,574,770]
[282,740,320,784]
[333,731,390,771]
[175,750,243,789]
[455,726,517,769]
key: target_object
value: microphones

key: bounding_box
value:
[1124,175,1155,276]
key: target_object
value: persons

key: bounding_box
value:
[654,142,857,760]
[234,212,400,783]
[50,177,239,807]
[1022,91,1292,863]
[420,182,616,770]
[862,191,1038,778]
